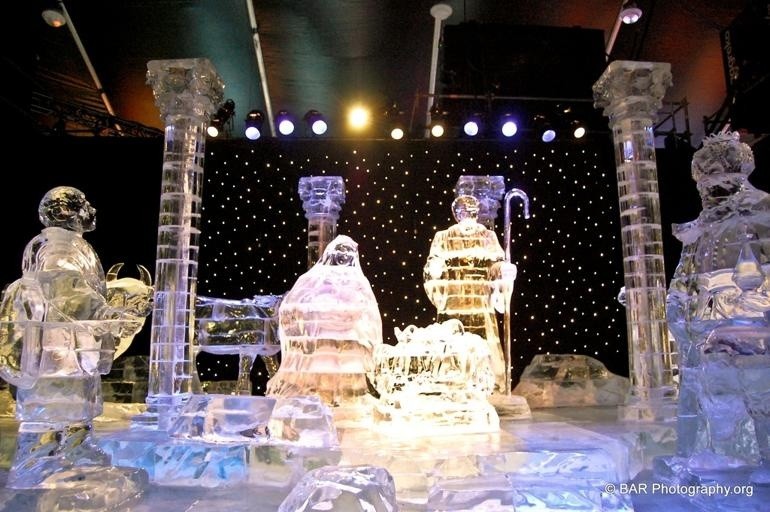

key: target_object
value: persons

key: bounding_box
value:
[9,188,143,463]
[656,122,770,498]
[422,195,518,392]
[265,236,385,423]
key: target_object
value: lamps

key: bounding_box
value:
[41,5,66,29]
[206,88,596,142]
[619,3,643,25]
[430,4,453,21]
